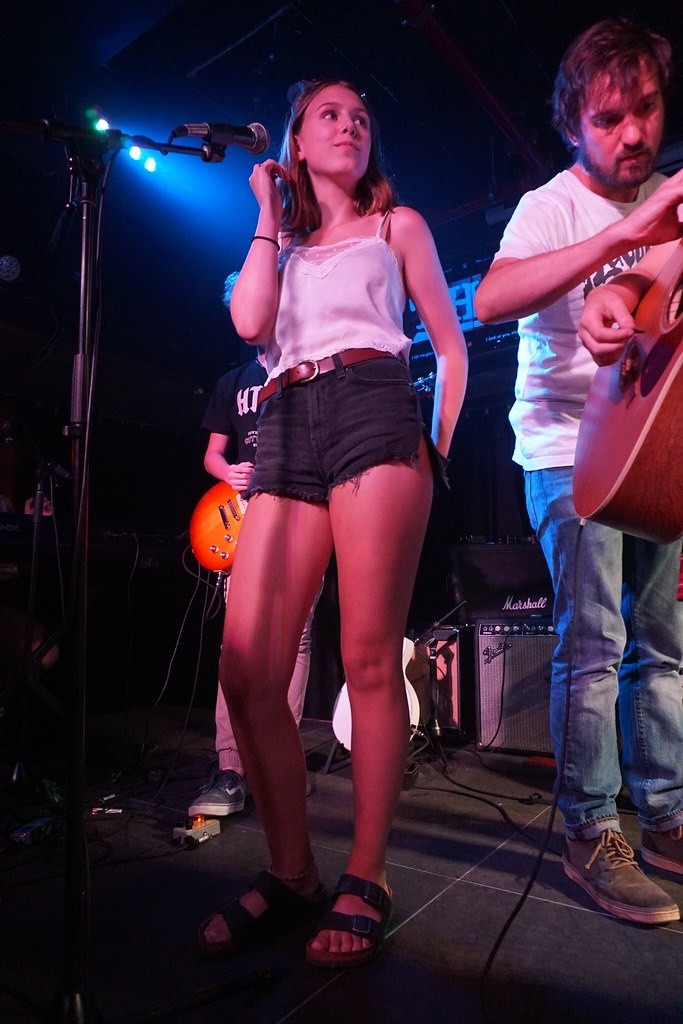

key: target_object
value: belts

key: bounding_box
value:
[257,343,398,403]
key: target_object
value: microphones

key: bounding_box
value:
[172,123,270,156]
[0,416,14,442]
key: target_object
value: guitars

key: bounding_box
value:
[190,369,435,573]
[331,636,422,756]
[573,239,682,544]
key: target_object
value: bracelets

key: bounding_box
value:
[251,236,279,250]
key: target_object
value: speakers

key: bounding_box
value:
[408,623,474,734]
[469,619,558,752]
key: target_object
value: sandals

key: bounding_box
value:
[307,873,394,967]
[201,866,327,950]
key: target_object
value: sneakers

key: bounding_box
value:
[641,825,683,872]
[561,829,682,924]
[189,768,251,814]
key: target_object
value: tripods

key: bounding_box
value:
[410,600,468,775]
[0,118,277,1023]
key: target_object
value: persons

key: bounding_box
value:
[580,233,683,367]
[472,18,682,923]
[189,351,325,816]
[0,415,59,674]
[203,79,468,968]
